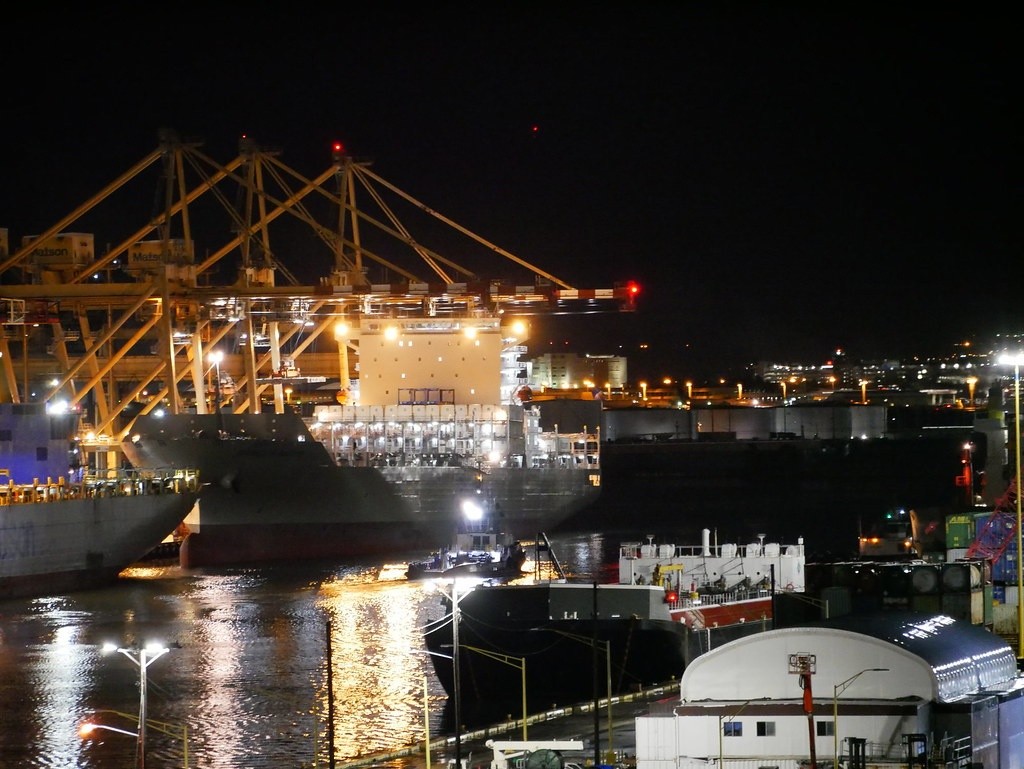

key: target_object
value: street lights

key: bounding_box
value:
[996,353,1024,662]
[83,708,189,769]
[399,574,482,769]
[79,641,170,769]
[336,660,433,769]
[531,626,617,765]
[775,588,829,620]
[832,668,890,769]
[719,696,772,769]
[439,641,529,743]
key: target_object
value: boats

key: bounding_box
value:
[0,400,206,602]
[422,523,809,722]
[118,306,607,568]
[404,500,529,579]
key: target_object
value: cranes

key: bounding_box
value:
[0,128,642,483]
